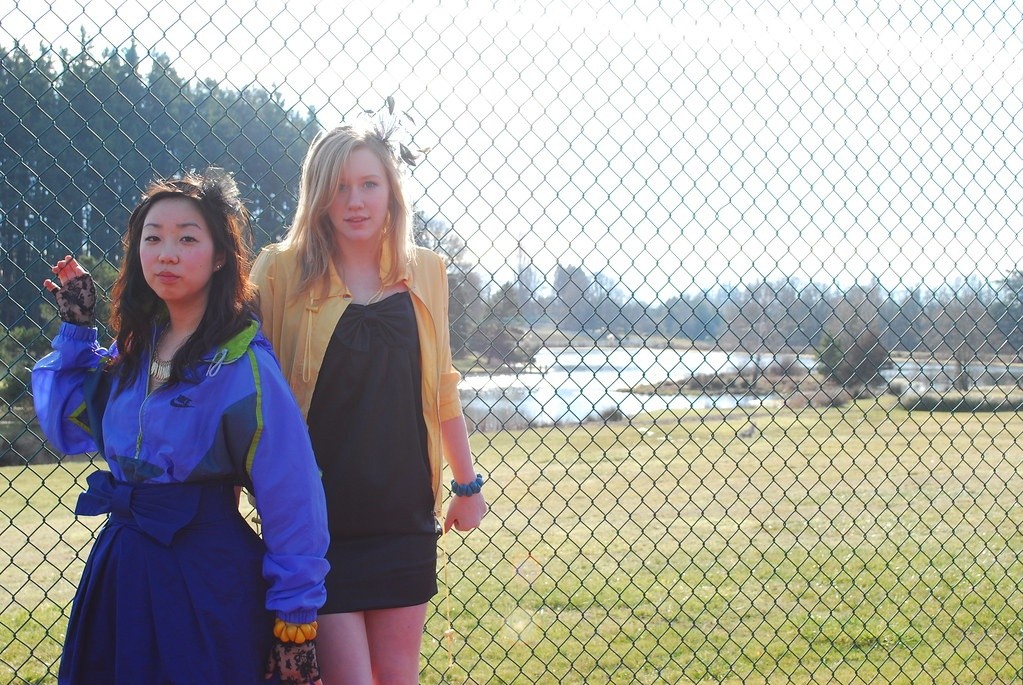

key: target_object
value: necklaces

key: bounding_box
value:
[366,286,384,306]
[150,323,172,379]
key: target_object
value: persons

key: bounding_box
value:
[248,124,487,685]
[32,174,330,685]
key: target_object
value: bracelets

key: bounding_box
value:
[273,618,318,643]
[451,474,483,497]
